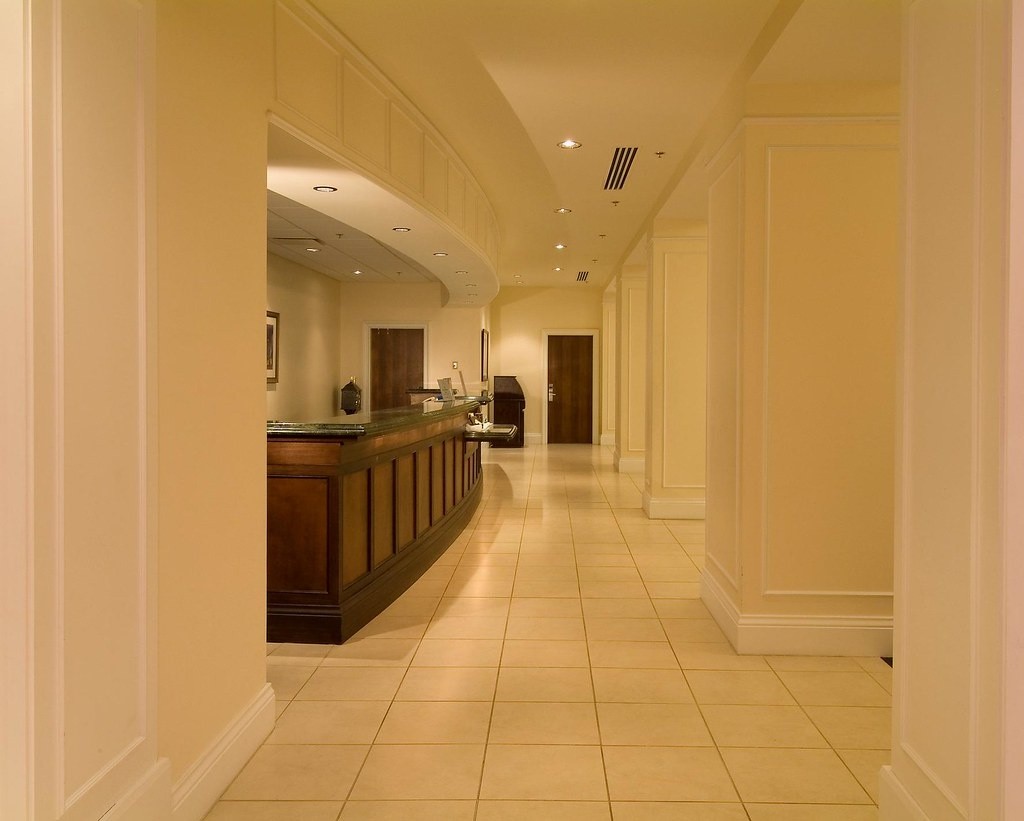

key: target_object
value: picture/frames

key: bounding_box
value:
[482,328,490,382]
[267,310,281,383]
